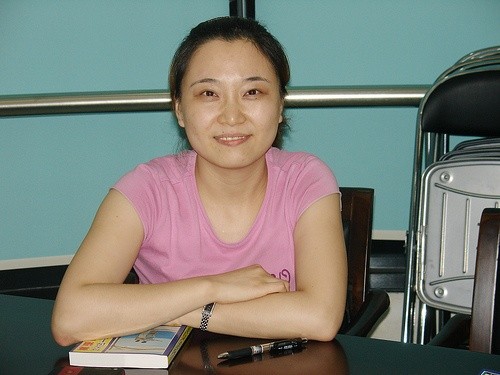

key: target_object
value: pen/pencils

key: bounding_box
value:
[217,337,309,360]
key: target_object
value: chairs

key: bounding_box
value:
[401,46,500,354]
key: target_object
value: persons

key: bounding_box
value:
[50,16,348,346]
[168,334,349,375]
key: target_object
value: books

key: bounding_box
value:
[67,324,193,369]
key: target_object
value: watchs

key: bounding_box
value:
[199,340,215,375]
[199,302,215,332]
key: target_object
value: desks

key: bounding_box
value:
[0,294,500,375]
